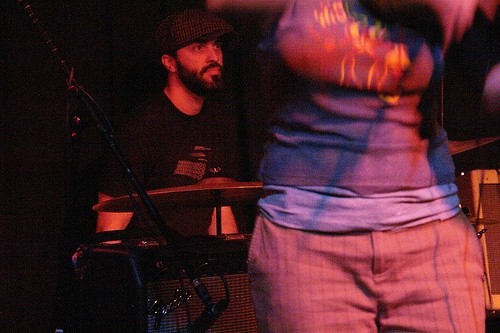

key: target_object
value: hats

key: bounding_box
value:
[154,10,234,53]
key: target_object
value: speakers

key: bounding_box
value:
[75,235,260,333]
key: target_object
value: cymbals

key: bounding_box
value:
[448,136,498,157]
[92,178,265,213]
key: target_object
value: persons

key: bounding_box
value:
[207,1,500,333]
[83,8,248,244]
